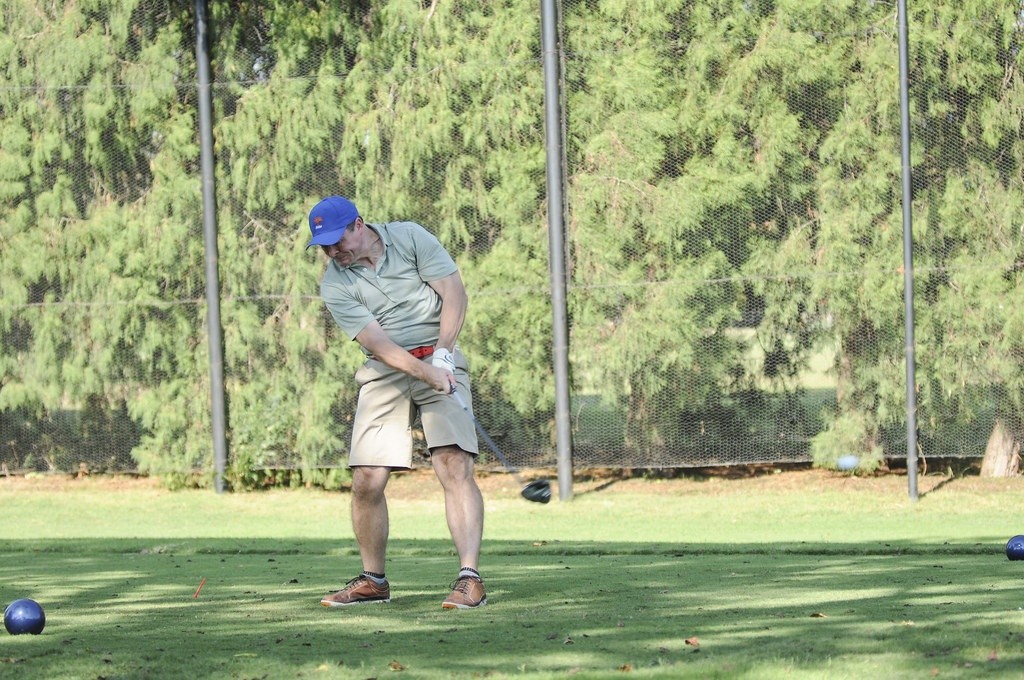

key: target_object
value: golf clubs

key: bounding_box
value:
[451,385,552,505]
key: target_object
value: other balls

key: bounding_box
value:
[1006,534,1024,561]
[4,598,46,636]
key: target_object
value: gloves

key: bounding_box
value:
[431,348,456,395]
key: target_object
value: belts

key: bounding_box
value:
[407,344,438,359]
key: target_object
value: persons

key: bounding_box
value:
[306,195,488,609]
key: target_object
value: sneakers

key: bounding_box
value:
[443,575,487,608]
[321,574,391,607]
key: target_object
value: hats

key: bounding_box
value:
[305,196,359,251]
